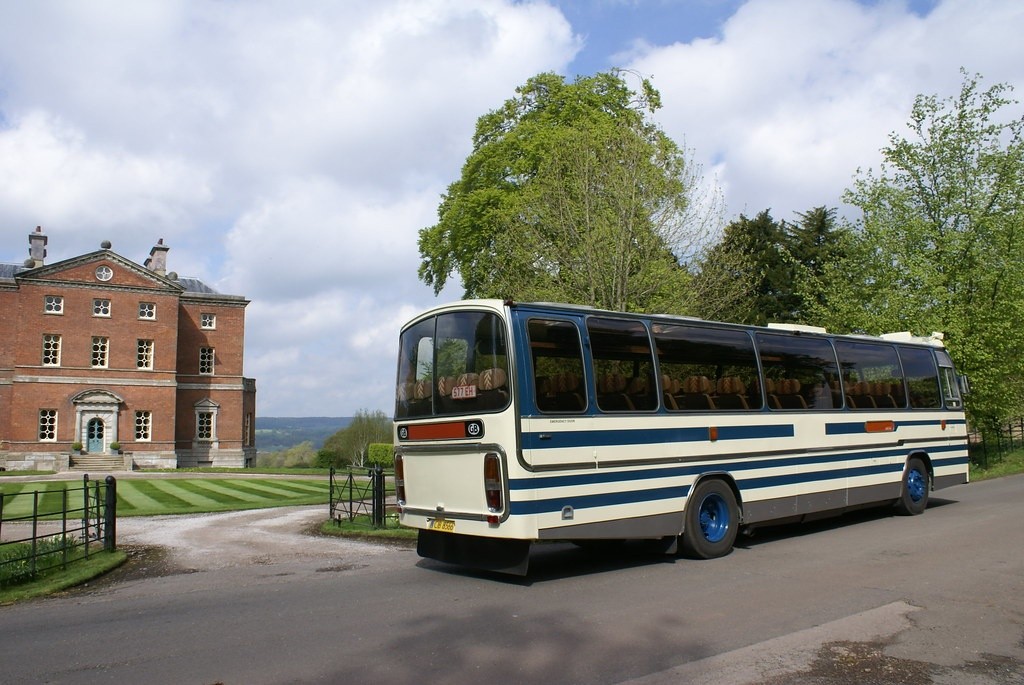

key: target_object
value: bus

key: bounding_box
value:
[393,301,970,576]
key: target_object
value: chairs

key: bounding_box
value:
[415,380,432,417]
[451,373,481,413]
[397,382,414,417]
[438,375,456,413]
[537,375,915,413]
[478,368,509,414]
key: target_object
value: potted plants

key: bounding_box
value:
[71,443,82,455]
[110,442,120,455]
